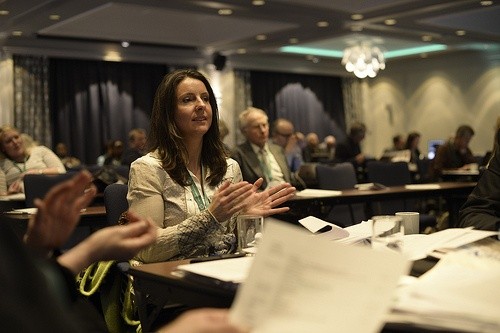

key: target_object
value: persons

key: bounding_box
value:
[270,120,474,181]
[229,108,292,190]
[0,125,148,195]
[127,69,296,263]
[455,118,500,240]
[0,171,250,333]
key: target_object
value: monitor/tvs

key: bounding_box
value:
[427,140,444,160]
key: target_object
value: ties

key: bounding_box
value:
[260,150,272,183]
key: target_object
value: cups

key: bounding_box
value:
[237,214,264,256]
[395,212,419,235]
[371,215,403,249]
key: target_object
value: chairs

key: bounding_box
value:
[289,154,442,224]
[24,173,73,208]
[104,183,133,273]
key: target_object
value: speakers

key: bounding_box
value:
[215,55,227,71]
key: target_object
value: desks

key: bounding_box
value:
[129,233,500,333]
[9,182,476,255]
